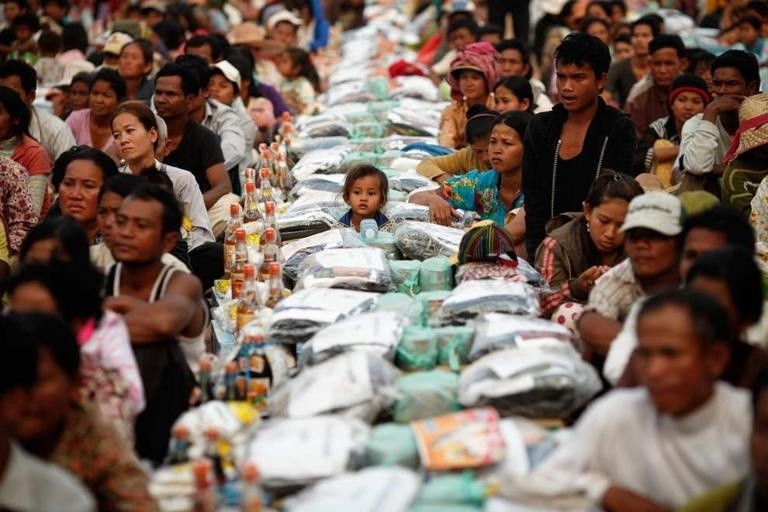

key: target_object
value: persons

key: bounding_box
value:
[408,1,767,511]
[1,1,368,512]
[338,165,391,232]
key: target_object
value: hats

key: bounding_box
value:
[209,59,241,91]
[617,192,685,237]
[451,64,481,79]
[457,221,518,268]
[735,93,768,158]
[668,73,711,106]
[101,31,133,55]
[267,10,304,30]
[51,61,95,88]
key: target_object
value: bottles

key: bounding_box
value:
[169,107,294,511]
